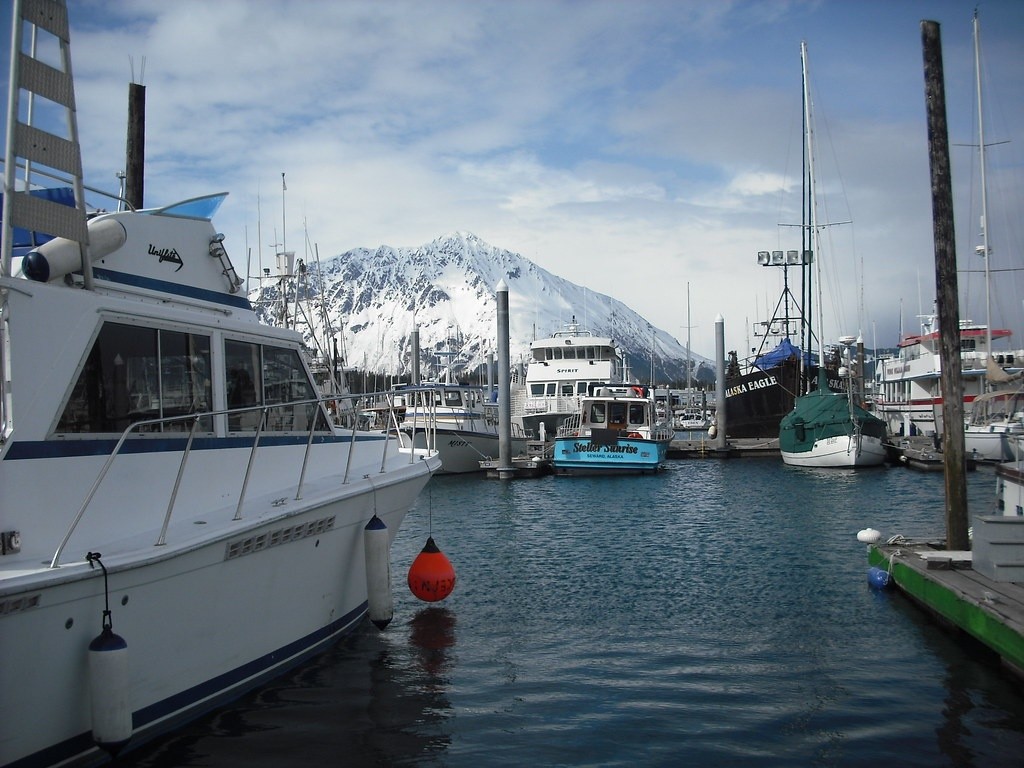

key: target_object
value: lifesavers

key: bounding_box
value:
[630,387,643,398]
[628,433,643,438]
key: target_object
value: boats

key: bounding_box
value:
[1,0,450,768]
[392,4,1024,481]
